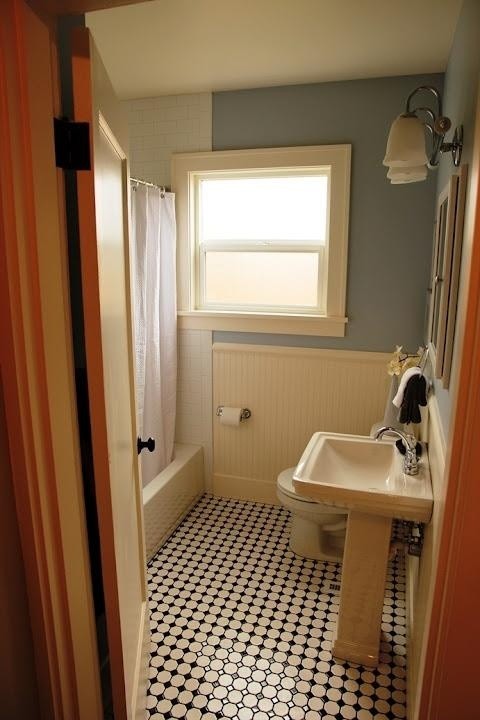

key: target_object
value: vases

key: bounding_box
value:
[383,373,400,429]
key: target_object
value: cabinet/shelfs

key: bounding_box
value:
[429,165,466,392]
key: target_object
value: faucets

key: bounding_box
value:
[374,427,419,476]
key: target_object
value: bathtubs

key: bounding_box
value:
[141,445,206,565]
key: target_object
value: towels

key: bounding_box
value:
[390,365,431,427]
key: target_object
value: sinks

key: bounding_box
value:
[291,431,434,511]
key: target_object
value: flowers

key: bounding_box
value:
[386,344,423,379]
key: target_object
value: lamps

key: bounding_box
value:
[381,84,465,186]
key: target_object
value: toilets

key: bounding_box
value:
[276,424,397,564]
